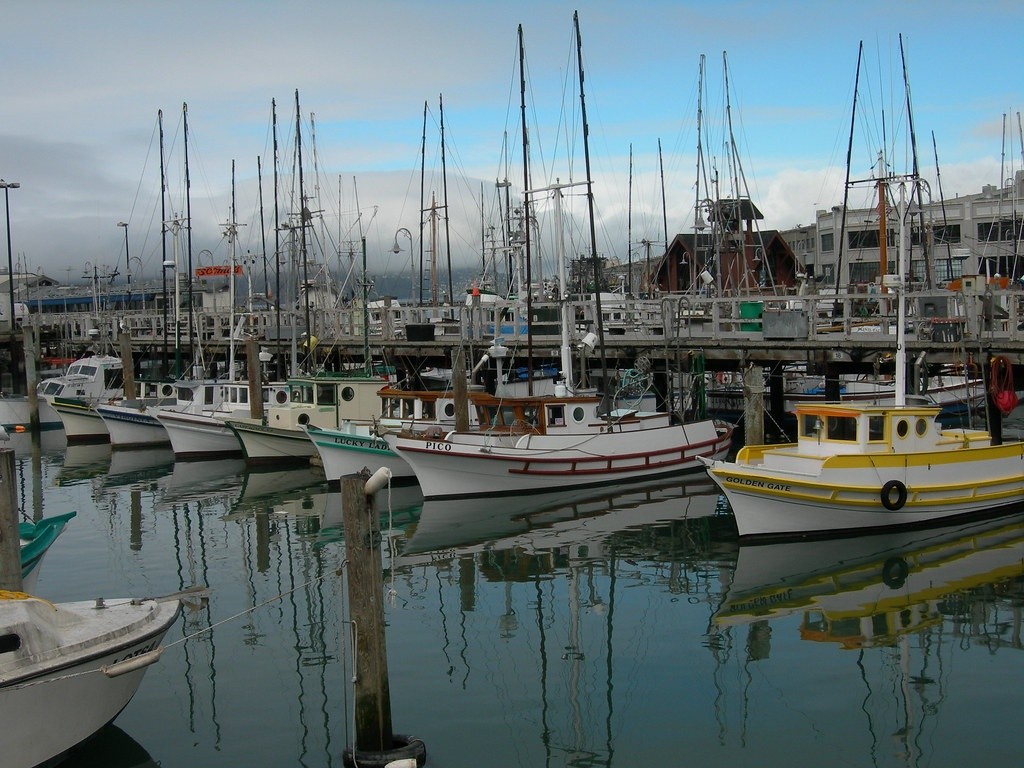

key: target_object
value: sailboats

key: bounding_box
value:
[2,429,1023,768]
[0,9,1024,538]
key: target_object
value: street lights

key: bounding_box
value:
[83,260,97,316]
[680,252,692,292]
[1004,229,1015,280]
[35,265,47,315]
[388,228,418,308]
[197,249,217,317]
[118,222,132,311]
[907,178,936,293]
[691,199,722,303]
[795,240,808,278]
[751,248,765,285]
[1,183,20,393]
[277,243,299,313]
[124,256,147,315]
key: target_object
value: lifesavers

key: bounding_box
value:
[881,556,910,589]
[880,480,908,511]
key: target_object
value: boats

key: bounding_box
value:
[0,590,214,768]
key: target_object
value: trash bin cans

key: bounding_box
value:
[739,301,764,332]
